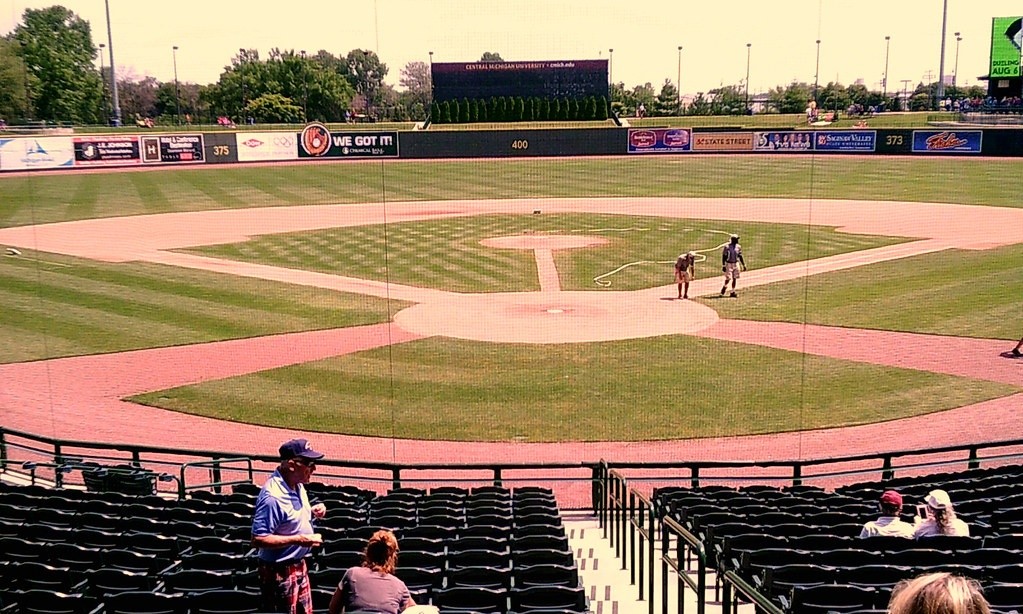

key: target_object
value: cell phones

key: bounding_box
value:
[917,504,928,518]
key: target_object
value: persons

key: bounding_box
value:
[329,530,418,614]
[721,234,748,298]
[889,573,991,614]
[861,490,914,536]
[252,438,326,614]
[916,489,970,536]
[674,251,696,298]
[1011,338,1023,357]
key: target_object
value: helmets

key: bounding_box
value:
[687,252,697,261]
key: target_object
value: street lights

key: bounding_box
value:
[173,46,180,125]
[240,49,246,108]
[429,52,433,62]
[952,32,962,86]
[99,44,109,124]
[745,43,751,108]
[609,49,613,92]
[814,40,821,102]
[363,52,368,116]
[677,46,682,112]
[884,36,890,99]
[20,41,31,119]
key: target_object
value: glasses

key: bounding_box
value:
[294,459,315,466]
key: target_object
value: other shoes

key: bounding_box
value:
[683,295,690,299]
[721,286,727,295]
[678,295,683,300]
[730,292,739,297]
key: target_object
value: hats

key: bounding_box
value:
[923,489,951,509]
[731,234,740,239]
[880,491,903,506]
[279,438,324,460]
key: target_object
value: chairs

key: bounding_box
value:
[0,463,1023,614]
